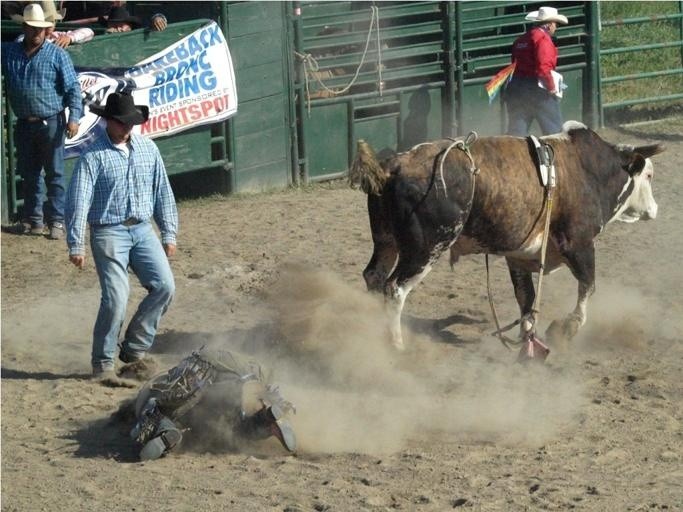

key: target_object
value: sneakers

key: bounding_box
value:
[93,371,119,382]
[48,222,66,239]
[264,405,297,452]
[138,430,181,461]
[30,224,45,235]
[119,347,145,364]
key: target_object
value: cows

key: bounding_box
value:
[345,119,668,354]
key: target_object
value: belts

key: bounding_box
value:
[90,217,142,227]
[18,115,45,124]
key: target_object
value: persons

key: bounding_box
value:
[504,6,571,137]
[0,1,220,49]
[114,343,301,460]
[0,4,84,240]
[63,91,182,383]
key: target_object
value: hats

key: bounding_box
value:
[89,92,150,126]
[98,7,137,25]
[525,6,569,25]
[36,1,67,22]
[12,3,54,28]
